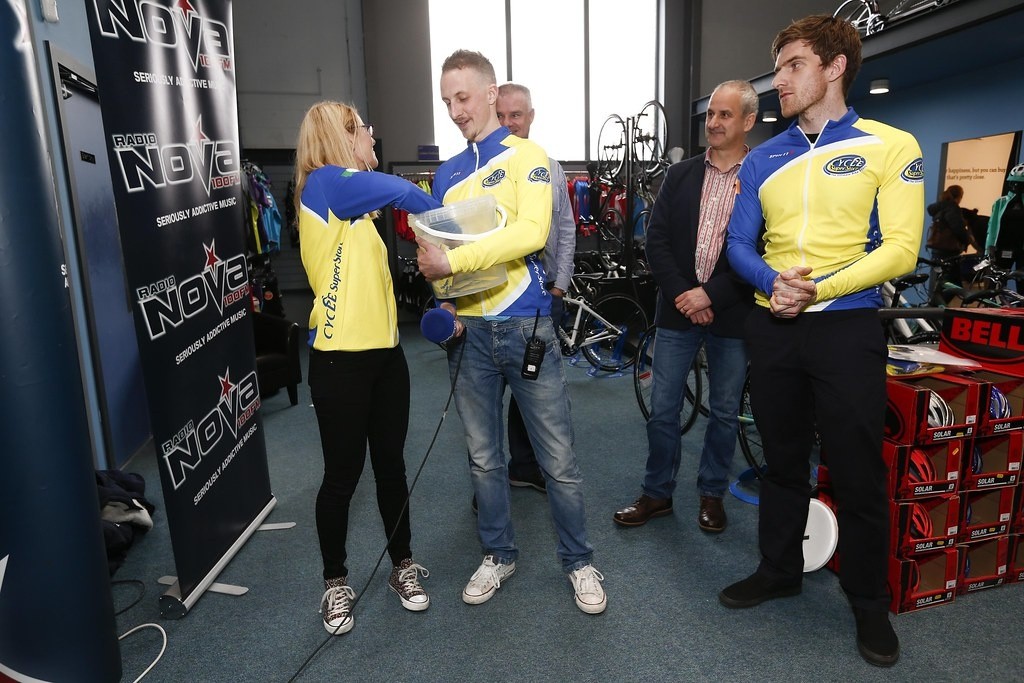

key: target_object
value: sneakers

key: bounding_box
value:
[462,555,515,604]
[853,596,900,667]
[720,569,802,608]
[319,577,356,635]
[568,563,607,613]
[388,558,429,611]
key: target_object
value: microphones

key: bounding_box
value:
[420,308,456,344]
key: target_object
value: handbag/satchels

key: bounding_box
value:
[925,203,958,250]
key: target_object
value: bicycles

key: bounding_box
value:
[880,254,1024,353]
[395,244,604,314]
[597,101,674,280]
[556,272,650,372]
[633,322,821,498]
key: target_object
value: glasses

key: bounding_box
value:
[351,124,373,136]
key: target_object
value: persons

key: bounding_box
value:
[985,162,1024,308]
[416,48,607,614]
[471,83,576,514]
[294,99,479,635]
[613,79,771,533]
[719,14,925,668]
[927,185,975,308]
[960,207,1015,289]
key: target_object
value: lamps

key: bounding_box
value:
[763,111,778,122]
[870,80,890,94]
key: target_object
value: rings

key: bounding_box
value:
[683,307,688,312]
[554,287,567,295]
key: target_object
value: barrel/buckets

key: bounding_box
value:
[408,194,508,299]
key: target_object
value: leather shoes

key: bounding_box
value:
[613,493,673,526]
[699,496,727,533]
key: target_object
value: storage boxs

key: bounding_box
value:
[818,307,1024,616]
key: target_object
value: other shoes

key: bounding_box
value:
[472,495,478,511]
[508,458,546,493]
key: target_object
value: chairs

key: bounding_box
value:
[929,254,1002,307]
[593,277,660,338]
[253,311,303,407]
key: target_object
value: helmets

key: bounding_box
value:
[989,386,1012,418]
[912,560,920,594]
[927,388,954,427]
[966,504,971,526]
[1007,163,1024,181]
[908,447,937,483]
[964,556,971,577]
[971,448,984,475]
[908,502,933,539]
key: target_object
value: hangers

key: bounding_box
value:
[393,168,628,193]
[400,256,426,283]
